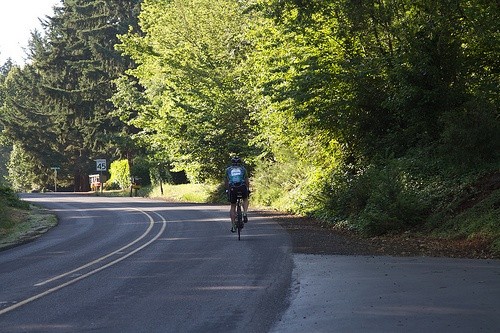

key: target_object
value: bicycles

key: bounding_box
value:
[225,183,250,239]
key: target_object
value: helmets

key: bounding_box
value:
[232,157,241,163]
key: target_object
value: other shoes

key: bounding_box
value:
[232,227,236,232]
[243,213,247,223]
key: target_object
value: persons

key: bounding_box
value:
[224,155,252,233]
